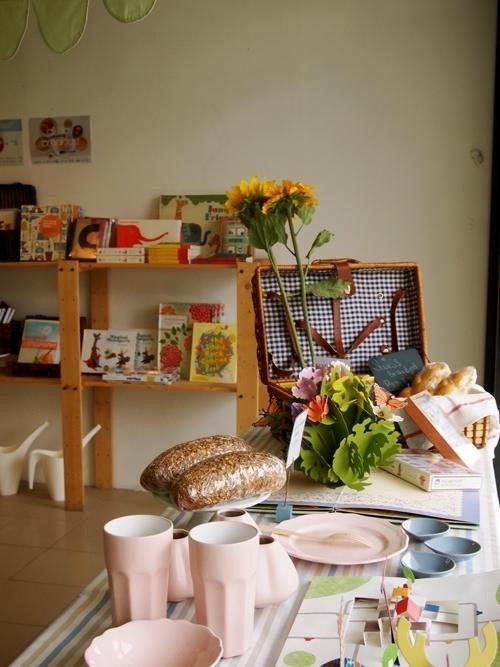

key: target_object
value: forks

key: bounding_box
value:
[264,525,378,549]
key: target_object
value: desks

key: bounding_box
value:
[2,411,500,667]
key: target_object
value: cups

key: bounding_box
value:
[188,520,259,659]
[26,421,108,502]
[100,515,174,624]
[0,420,48,497]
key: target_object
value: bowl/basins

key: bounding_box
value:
[85,616,225,666]
[35,117,86,152]
[402,517,482,576]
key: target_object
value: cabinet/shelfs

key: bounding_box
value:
[0,254,274,514]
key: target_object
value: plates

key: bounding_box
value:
[272,512,409,564]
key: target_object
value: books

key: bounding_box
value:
[382,447,483,491]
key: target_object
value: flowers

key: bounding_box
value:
[224,177,344,382]
[291,367,402,490]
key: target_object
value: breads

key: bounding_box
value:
[398,361,477,399]
[139,435,252,495]
[174,451,286,510]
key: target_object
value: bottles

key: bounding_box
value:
[209,509,299,605]
[167,528,194,604]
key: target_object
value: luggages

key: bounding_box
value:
[248,259,493,454]
